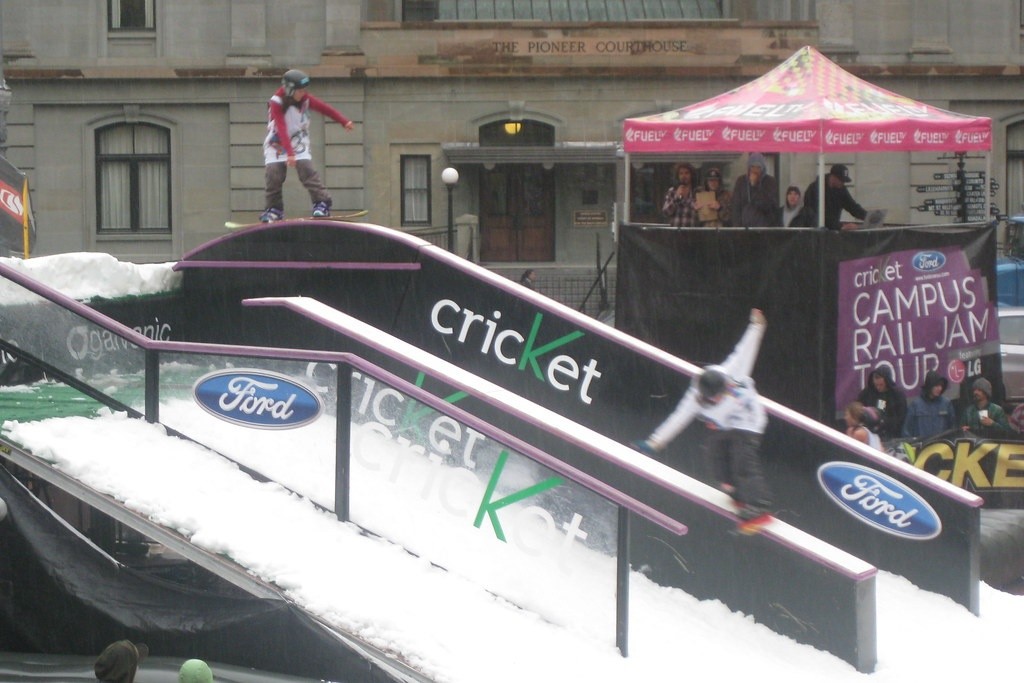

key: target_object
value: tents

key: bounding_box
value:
[621,45,994,230]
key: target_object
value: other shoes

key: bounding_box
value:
[737,513,771,535]
[720,482,737,493]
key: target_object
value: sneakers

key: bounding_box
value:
[314,202,330,217]
[262,208,283,221]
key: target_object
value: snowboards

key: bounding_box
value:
[224,209,370,229]
[720,482,771,537]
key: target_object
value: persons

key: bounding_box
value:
[843,365,1009,451]
[259,71,354,224]
[661,151,805,227]
[629,307,773,532]
[94,639,149,683]
[803,164,868,230]
[519,269,537,289]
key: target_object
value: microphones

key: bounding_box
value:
[679,181,685,199]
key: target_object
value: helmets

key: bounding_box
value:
[284,70,309,89]
[861,406,881,432]
[699,370,724,396]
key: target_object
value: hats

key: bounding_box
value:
[831,164,852,182]
[935,379,944,389]
[704,168,722,179]
[973,377,992,398]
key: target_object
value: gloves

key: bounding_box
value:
[635,441,655,456]
[750,308,764,323]
